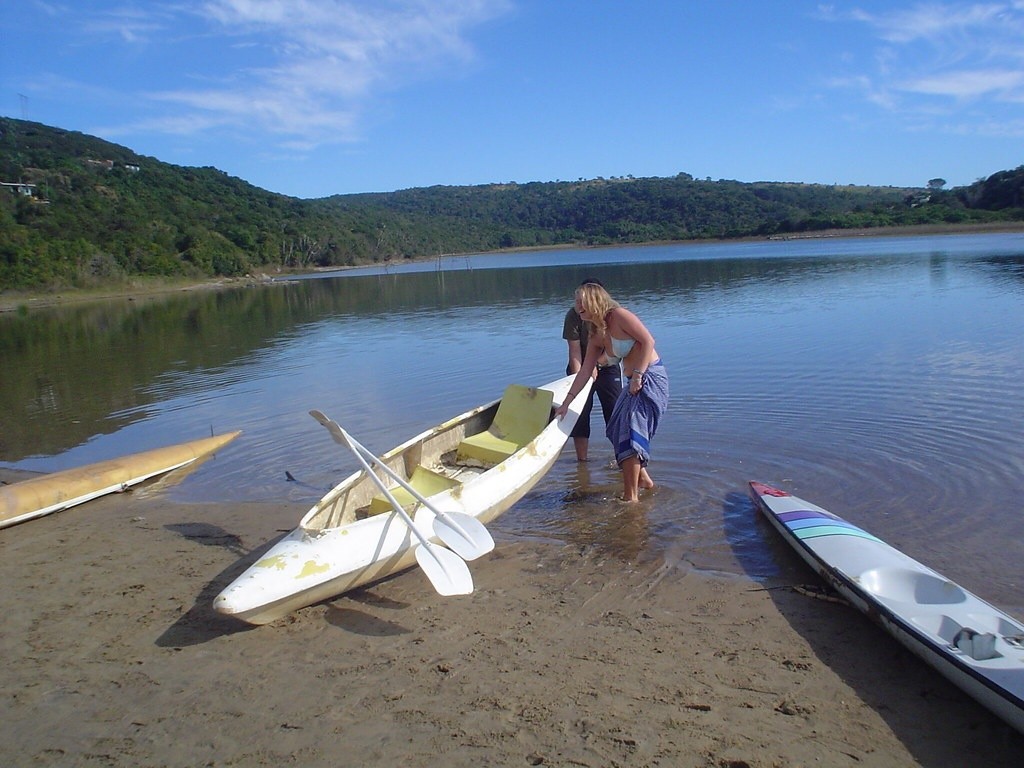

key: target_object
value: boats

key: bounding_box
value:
[213,366,595,623]
[747,478,1024,736]
[0,428,243,530]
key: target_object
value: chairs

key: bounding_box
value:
[457,384,554,464]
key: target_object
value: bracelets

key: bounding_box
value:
[596,361,602,370]
[633,369,643,375]
[632,378,642,382]
[568,393,575,399]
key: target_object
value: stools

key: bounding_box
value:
[368,465,465,518]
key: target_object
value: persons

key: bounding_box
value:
[562,278,622,462]
[553,283,668,500]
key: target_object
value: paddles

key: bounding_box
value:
[308,409,495,562]
[318,419,474,597]
